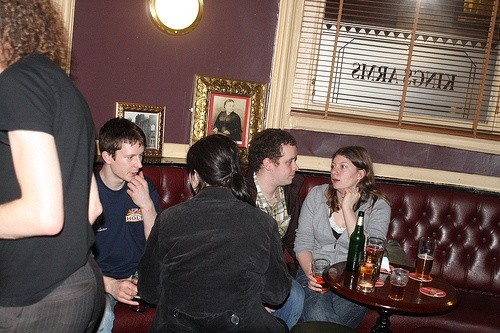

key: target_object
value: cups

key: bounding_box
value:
[388,268,410,301]
[311,258,331,294]
[357,251,378,293]
[366,236,385,277]
[130,270,148,312]
[415,236,437,280]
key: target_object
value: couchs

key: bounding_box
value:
[92,164,500,333]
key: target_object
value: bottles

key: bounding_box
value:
[346,211,366,272]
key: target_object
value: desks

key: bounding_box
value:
[322,262,460,333]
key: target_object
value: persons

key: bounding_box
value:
[294,146,392,329]
[0,0,106,333]
[138,134,291,333]
[214,99,243,141]
[87,119,162,333]
[246,128,305,331]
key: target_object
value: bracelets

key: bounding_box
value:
[305,272,314,276]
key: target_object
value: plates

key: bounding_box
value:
[419,287,446,298]
[358,278,384,287]
[380,265,394,273]
[407,273,433,282]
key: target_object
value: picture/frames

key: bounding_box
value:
[188,75,267,167]
[115,102,166,163]
